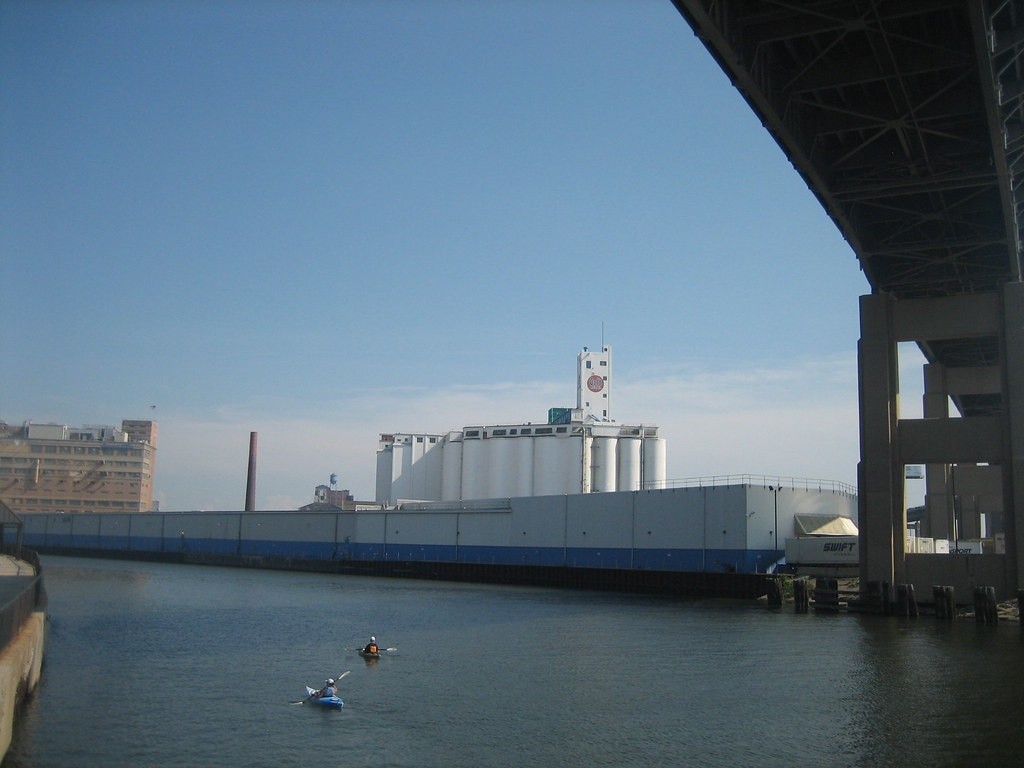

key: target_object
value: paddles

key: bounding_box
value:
[344,647,398,654]
[289,670,351,706]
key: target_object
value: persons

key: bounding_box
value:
[315,679,337,698]
[364,636,379,655]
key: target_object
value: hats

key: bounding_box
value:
[325,679,334,685]
[370,637,375,643]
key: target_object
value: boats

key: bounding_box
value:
[305,686,344,710]
[359,648,382,660]
